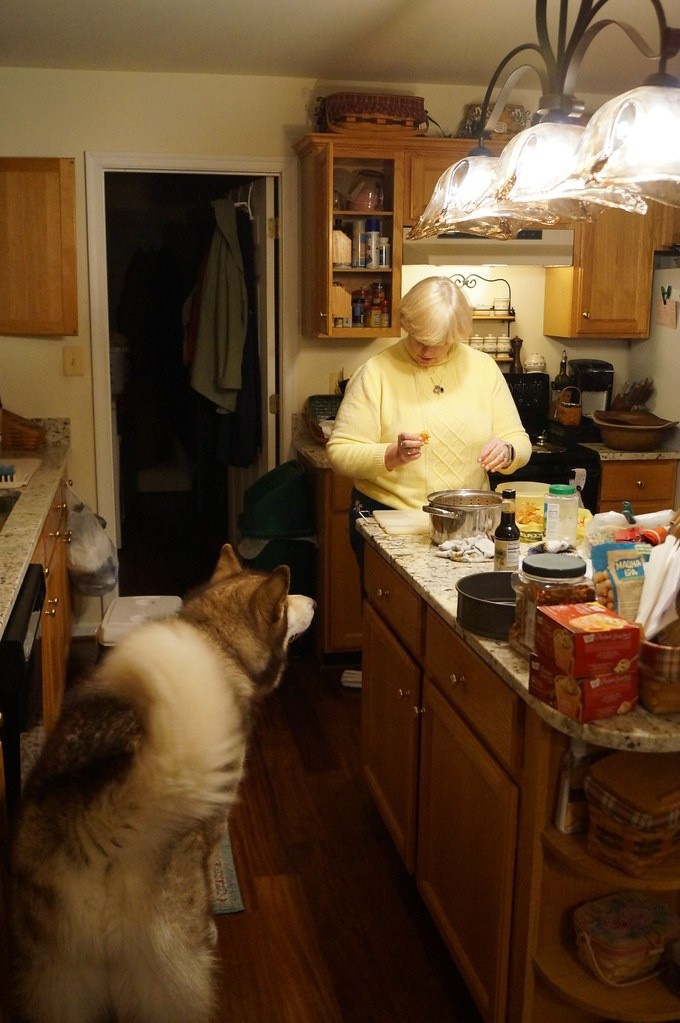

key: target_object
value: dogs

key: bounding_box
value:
[13,542,317,1023]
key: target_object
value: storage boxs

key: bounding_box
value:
[528,601,641,724]
[96,595,182,668]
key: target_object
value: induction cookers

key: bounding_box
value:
[489,374,601,462]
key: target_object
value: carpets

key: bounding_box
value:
[206,820,244,915]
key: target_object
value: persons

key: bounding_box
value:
[325,276,533,603]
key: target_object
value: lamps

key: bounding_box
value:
[405,0,680,241]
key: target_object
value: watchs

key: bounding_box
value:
[505,443,514,467]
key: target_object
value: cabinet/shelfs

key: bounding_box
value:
[354,535,680,1023]
[0,157,79,335]
[309,460,680,654]
[445,274,515,362]
[292,132,680,340]
[28,460,74,739]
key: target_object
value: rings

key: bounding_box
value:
[501,453,505,458]
[407,452,410,455]
[401,442,402,447]
[504,458,507,462]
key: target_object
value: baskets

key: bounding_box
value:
[555,387,582,427]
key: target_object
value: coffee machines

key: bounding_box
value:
[567,361,614,442]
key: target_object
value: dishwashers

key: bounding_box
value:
[0,562,44,806]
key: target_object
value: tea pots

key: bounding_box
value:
[348,168,389,212]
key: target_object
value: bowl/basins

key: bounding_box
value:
[592,409,679,451]
[455,569,518,640]
[495,481,550,540]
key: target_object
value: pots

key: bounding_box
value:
[421,490,502,545]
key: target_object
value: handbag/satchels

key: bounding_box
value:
[66,481,119,596]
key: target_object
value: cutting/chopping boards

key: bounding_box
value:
[373,510,431,535]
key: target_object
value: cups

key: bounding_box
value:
[494,297,510,316]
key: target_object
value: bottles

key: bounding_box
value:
[494,488,520,571]
[542,484,579,546]
[463,333,510,359]
[523,352,546,374]
[332,281,391,329]
[509,336,523,374]
[552,351,568,397]
[332,218,390,269]
[507,553,596,660]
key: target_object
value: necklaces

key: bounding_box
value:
[426,361,446,393]
